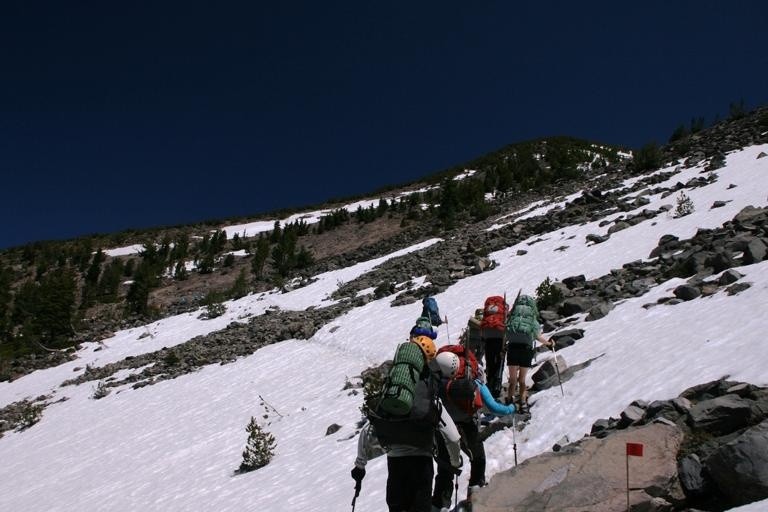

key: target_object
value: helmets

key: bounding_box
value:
[411,336,437,359]
[434,350,459,377]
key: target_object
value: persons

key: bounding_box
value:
[505,322,555,402]
[440,361,530,512]
[409,297,442,340]
[351,398,460,512]
[466,309,505,400]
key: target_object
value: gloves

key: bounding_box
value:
[351,466,366,482]
[514,399,529,415]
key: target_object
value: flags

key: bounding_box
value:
[626,442,646,457]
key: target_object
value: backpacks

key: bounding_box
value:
[435,343,482,413]
[364,357,443,447]
[479,296,507,339]
[506,294,540,345]
